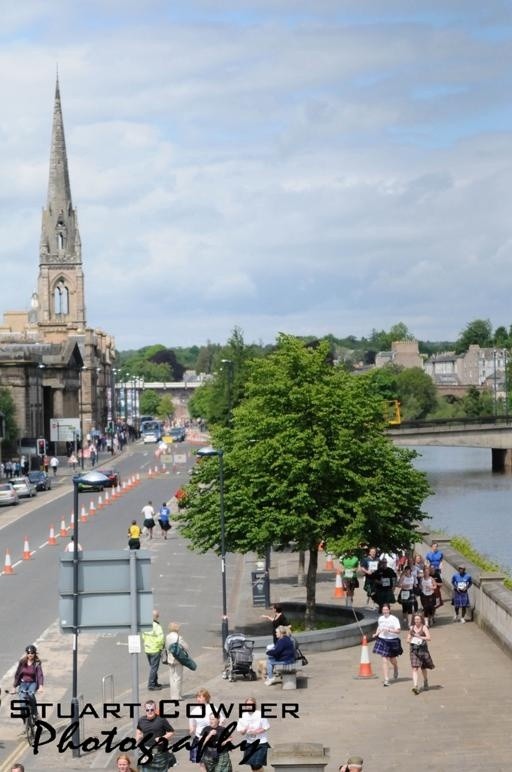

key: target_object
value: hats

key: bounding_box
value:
[347,756,363,769]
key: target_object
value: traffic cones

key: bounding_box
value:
[318,539,325,551]
[70,481,120,530]
[60,513,68,536]
[1,546,15,575]
[47,521,58,545]
[324,550,337,571]
[353,634,379,681]
[21,534,33,562]
[332,569,348,601]
[120,463,168,497]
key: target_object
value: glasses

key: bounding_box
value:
[146,709,155,712]
[27,652,34,654]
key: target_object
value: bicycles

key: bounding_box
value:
[6,690,44,747]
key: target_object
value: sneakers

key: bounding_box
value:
[461,617,466,624]
[264,676,276,686]
[453,615,459,621]
[424,680,429,690]
[412,687,421,695]
[393,673,398,679]
[149,684,163,690]
[384,679,392,686]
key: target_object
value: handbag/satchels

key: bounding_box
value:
[295,648,308,666]
[168,642,197,671]
[160,648,169,665]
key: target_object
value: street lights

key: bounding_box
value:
[95,367,143,439]
[492,349,498,416]
[34,363,46,438]
[79,366,87,470]
[502,347,508,427]
[70,472,113,759]
[196,447,230,662]
[220,357,233,428]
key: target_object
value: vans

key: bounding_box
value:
[8,477,36,498]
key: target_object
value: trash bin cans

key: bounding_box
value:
[252,571,269,607]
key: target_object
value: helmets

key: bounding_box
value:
[26,645,37,652]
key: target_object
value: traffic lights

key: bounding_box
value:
[36,439,46,456]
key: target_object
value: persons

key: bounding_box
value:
[116,688,271,771]
[65,535,82,552]
[11,763,24,772]
[127,519,143,550]
[141,608,165,690]
[265,535,471,694]
[158,502,171,540]
[141,501,156,541]
[338,757,364,772]
[163,622,192,701]
[13,645,44,732]
[0,413,137,480]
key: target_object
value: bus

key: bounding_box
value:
[142,420,162,440]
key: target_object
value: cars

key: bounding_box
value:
[27,470,50,491]
[170,427,186,442]
[143,432,159,444]
[97,467,119,487]
[0,483,18,506]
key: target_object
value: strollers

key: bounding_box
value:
[221,632,258,683]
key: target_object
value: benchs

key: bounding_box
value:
[272,655,305,691]
